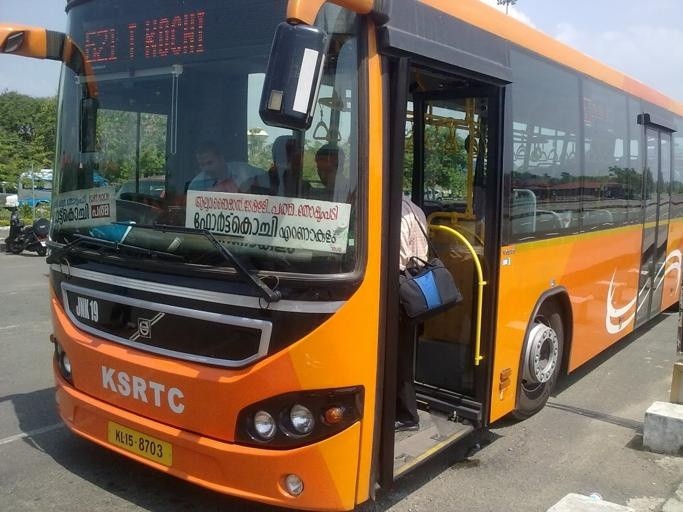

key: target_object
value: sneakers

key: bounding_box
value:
[392,417,420,432]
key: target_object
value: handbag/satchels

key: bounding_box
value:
[394,255,465,322]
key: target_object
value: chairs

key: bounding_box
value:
[421,187,614,235]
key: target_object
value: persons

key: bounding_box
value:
[312,143,355,232]
[239,134,315,199]
[185,137,266,199]
[391,189,428,433]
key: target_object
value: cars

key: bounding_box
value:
[424,190,442,201]
[0,180,19,206]
[113,175,166,200]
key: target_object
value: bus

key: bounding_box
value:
[19,167,109,207]
[0,0,683,512]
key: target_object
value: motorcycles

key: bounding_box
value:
[5,206,48,257]
